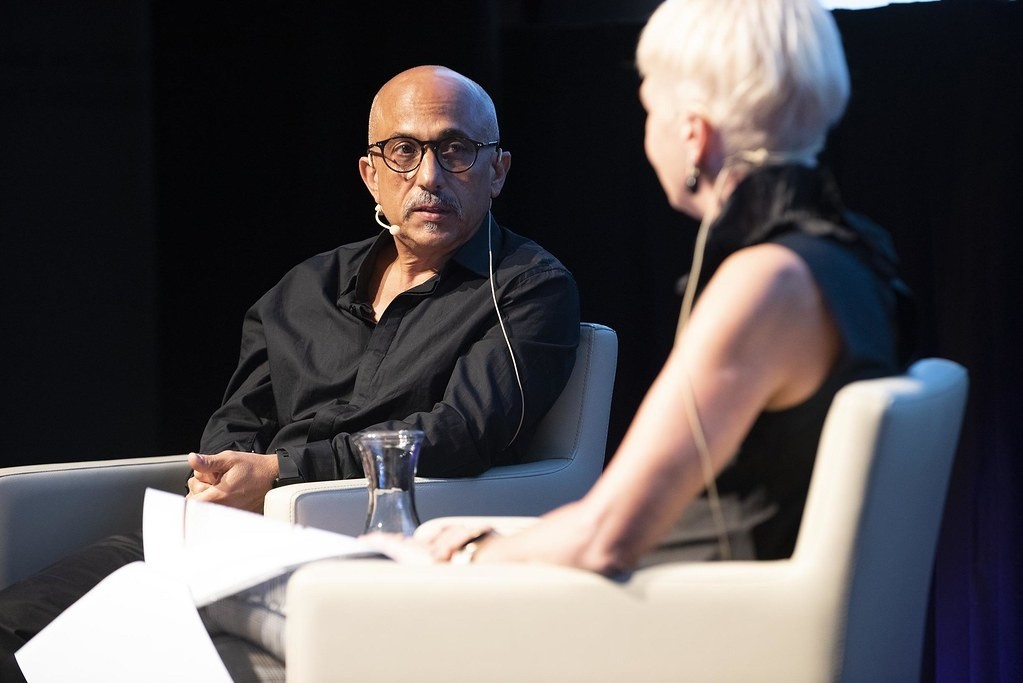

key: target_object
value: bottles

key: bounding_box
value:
[353,430,421,535]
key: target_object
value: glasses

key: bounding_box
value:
[368,136,498,174]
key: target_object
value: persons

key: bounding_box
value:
[414,0,911,578]
[0,65,584,683]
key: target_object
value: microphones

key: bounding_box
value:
[376,204,402,236]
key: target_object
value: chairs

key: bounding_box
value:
[0,322,619,584]
[284,355,970,682]
